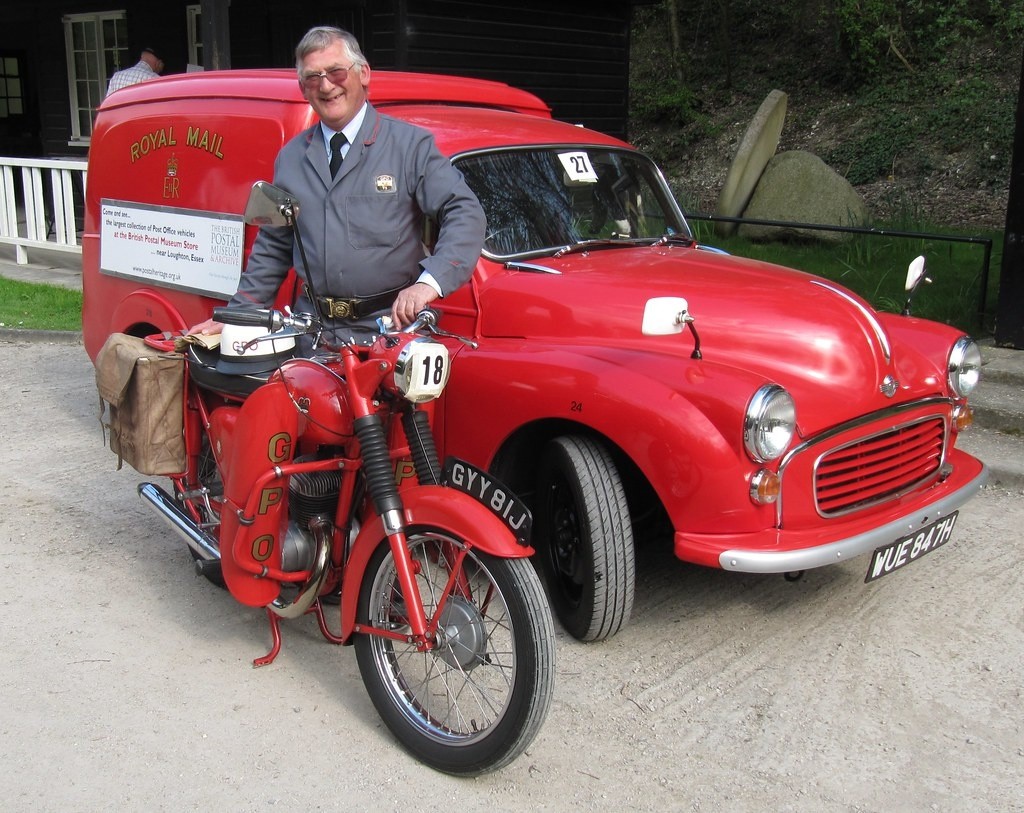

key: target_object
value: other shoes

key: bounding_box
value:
[322,589,342,604]
[390,603,407,616]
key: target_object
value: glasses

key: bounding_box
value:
[301,63,356,88]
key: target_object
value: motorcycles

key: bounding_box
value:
[134,183,555,781]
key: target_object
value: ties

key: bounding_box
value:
[329,132,348,182]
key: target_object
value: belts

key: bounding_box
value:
[303,277,418,324]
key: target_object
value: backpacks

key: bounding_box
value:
[95,333,185,475]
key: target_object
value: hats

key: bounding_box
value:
[215,309,296,374]
[141,39,169,72]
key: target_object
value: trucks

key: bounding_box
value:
[80,67,993,644]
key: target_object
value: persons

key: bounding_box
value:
[183,26,487,643]
[105,36,177,100]
